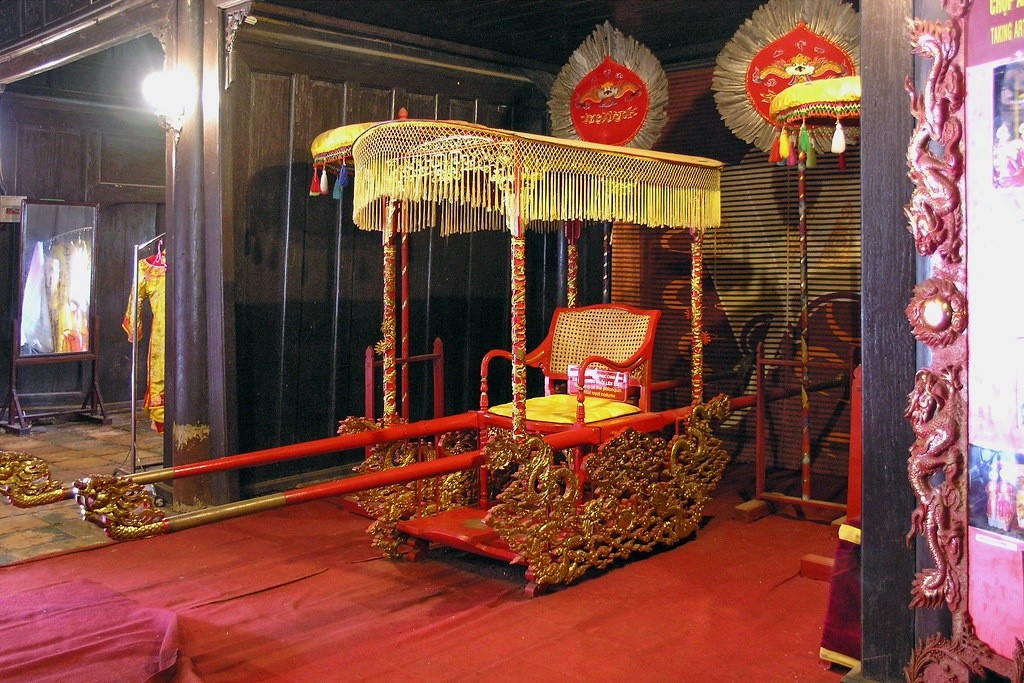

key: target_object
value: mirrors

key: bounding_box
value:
[0,198,113,436]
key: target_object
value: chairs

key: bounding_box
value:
[479,304,662,511]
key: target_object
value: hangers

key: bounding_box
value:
[146,238,167,272]
[46,229,86,253]
